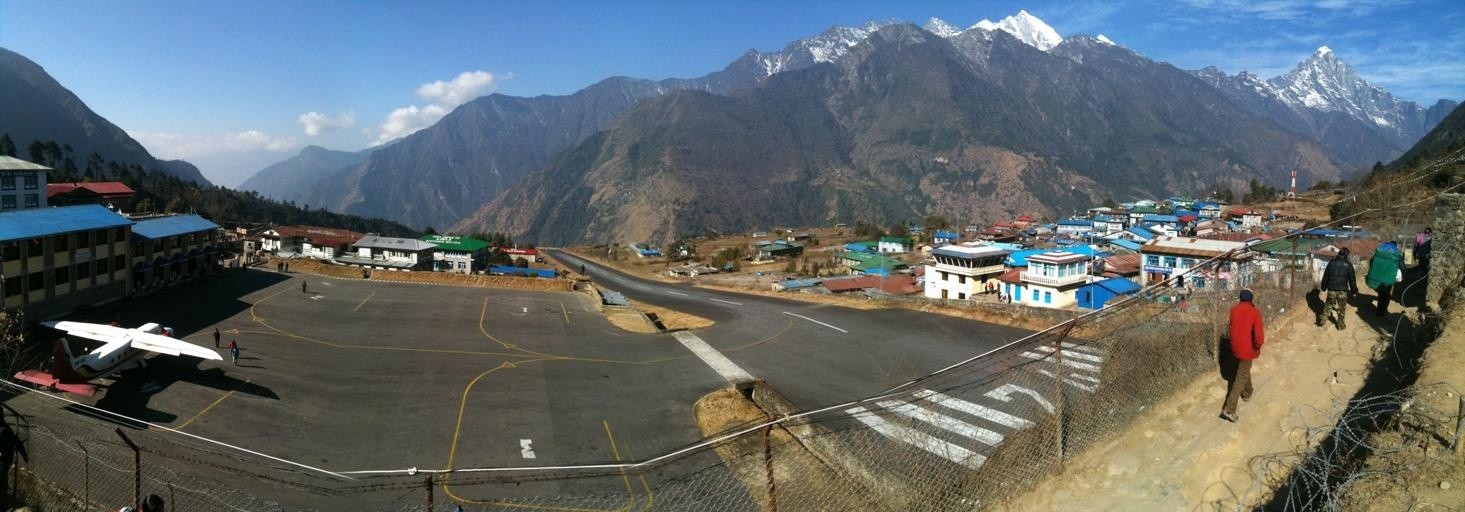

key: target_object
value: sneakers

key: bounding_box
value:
[1221,409,1239,422]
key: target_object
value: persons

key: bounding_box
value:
[1370,241,1406,315]
[278,261,289,273]
[119,495,164,511]
[219,254,246,272]
[1318,246,1358,330]
[227,339,240,365]
[214,328,221,348]
[0,407,28,509]
[1222,288,1263,423]
[1414,228,1432,278]
[302,281,306,293]
[580,264,585,276]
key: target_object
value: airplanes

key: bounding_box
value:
[39,320,224,380]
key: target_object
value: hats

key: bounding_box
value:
[1240,289,1254,301]
